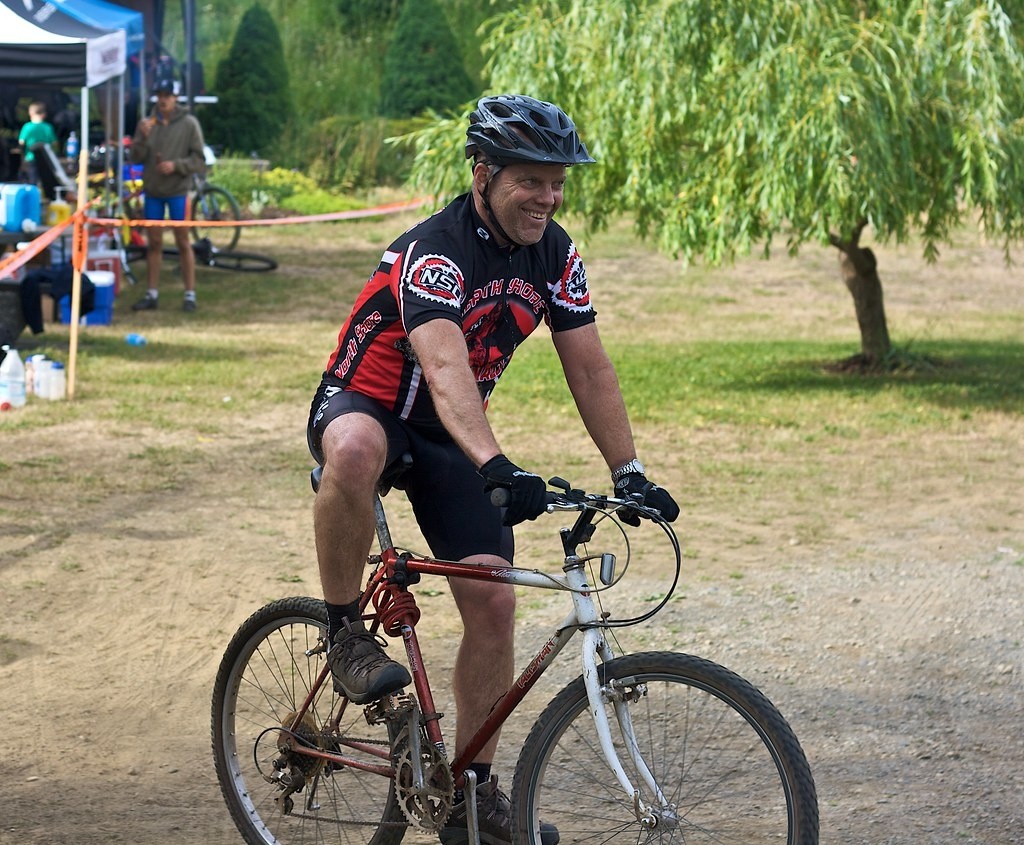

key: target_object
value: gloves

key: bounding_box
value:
[476,454,548,527]
[614,474,680,528]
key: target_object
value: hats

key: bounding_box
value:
[154,80,181,96]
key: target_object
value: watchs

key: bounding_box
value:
[611,458,644,483]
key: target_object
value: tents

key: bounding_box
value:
[0,1,144,400]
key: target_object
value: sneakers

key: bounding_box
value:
[326,616,411,706]
[433,773,560,845]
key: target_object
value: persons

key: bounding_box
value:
[128,78,206,311]
[18,100,57,186]
[307,94,681,845]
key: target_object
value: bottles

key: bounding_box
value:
[0,345,27,408]
[125,333,146,349]
[66,131,78,156]
[24,354,66,402]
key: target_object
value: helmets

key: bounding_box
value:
[465,94,598,163]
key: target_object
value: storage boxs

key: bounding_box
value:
[56,270,116,325]
[83,250,120,297]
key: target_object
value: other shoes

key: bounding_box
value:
[183,298,197,313]
[132,298,158,310]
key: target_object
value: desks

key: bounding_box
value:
[0,224,72,244]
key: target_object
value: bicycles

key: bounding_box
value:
[207,443,823,845]
[121,237,277,271]
[121,144,242,254]
